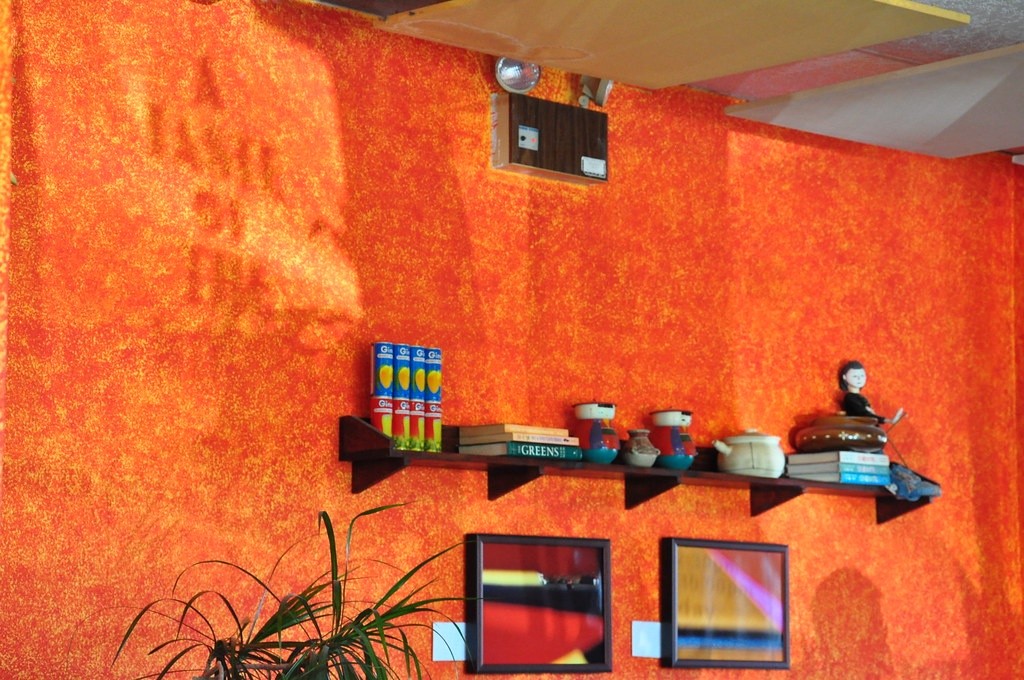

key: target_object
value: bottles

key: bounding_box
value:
[623,429,660,468]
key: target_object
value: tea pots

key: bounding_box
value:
[712,428,785,478]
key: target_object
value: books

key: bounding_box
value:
[787,450,892,486]
[456,421,584,460]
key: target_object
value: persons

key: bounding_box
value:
[837,360,885,424]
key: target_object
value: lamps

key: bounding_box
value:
[496,55,541,95]
[577,75,613,109]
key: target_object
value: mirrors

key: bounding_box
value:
[460,532,615,674]
[662,536,792,671]
